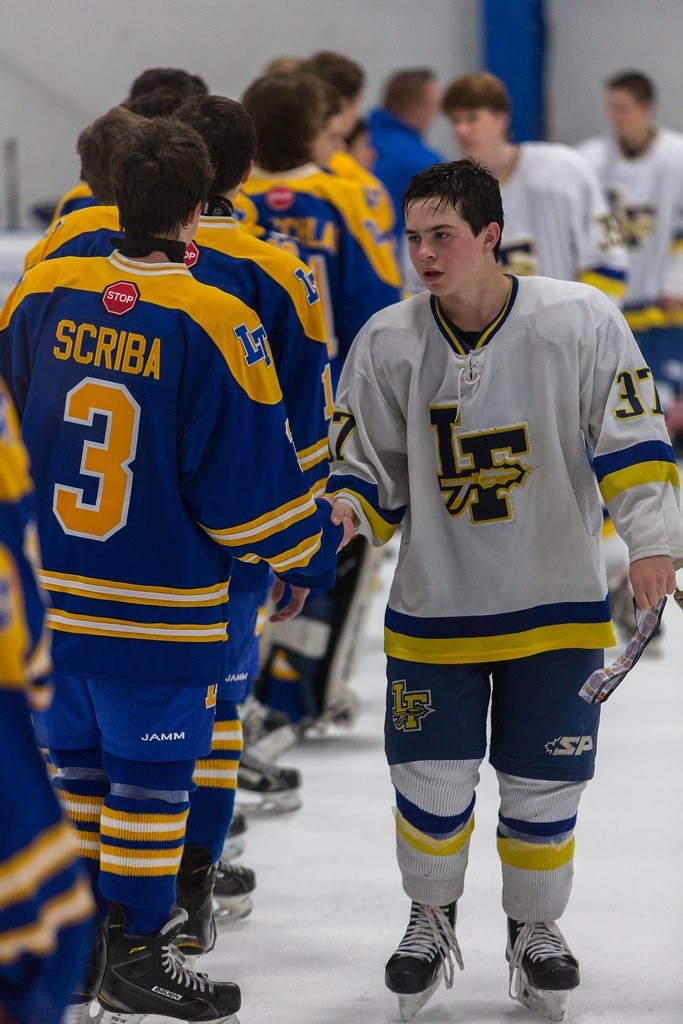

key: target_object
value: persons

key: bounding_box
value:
[25,51,405,970]
[327,161,682,1024]
[0,118,354,1024]
[576,68,683,444]
[363,70,447,299]
[432,73,663,647]
[0,378,99,1024]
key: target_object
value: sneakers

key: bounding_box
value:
[222,809,247,860]
[213,859,256,924]
[175,839,213,967]
[233,750,301,813]
[505,915,579,1021]
[385,900,464,1022]
[97,904,242,1023]
